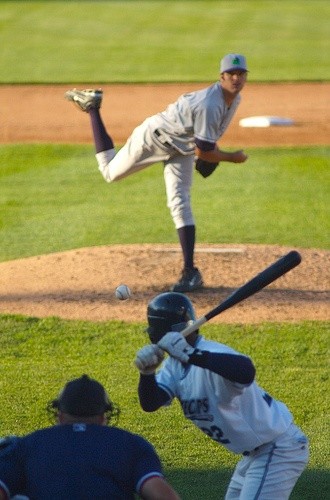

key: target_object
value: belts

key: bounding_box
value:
[155,129,175,150]
[242,448,260,456]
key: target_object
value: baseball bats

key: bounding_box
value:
[136,251,302,370]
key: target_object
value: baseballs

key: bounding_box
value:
[114,284,131,300]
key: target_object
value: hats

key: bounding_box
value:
[56,374,113,416]
[221,55,249,73]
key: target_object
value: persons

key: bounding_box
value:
[0,374,182,500]
[133,292,308,500]
[65,55,251,292]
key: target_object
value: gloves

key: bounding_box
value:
[158,331,197,364]
[134,344,164,375]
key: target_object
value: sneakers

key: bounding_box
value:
[65,87,104,111]
[172,267,203,291]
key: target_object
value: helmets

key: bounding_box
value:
[147,291,196,344]
